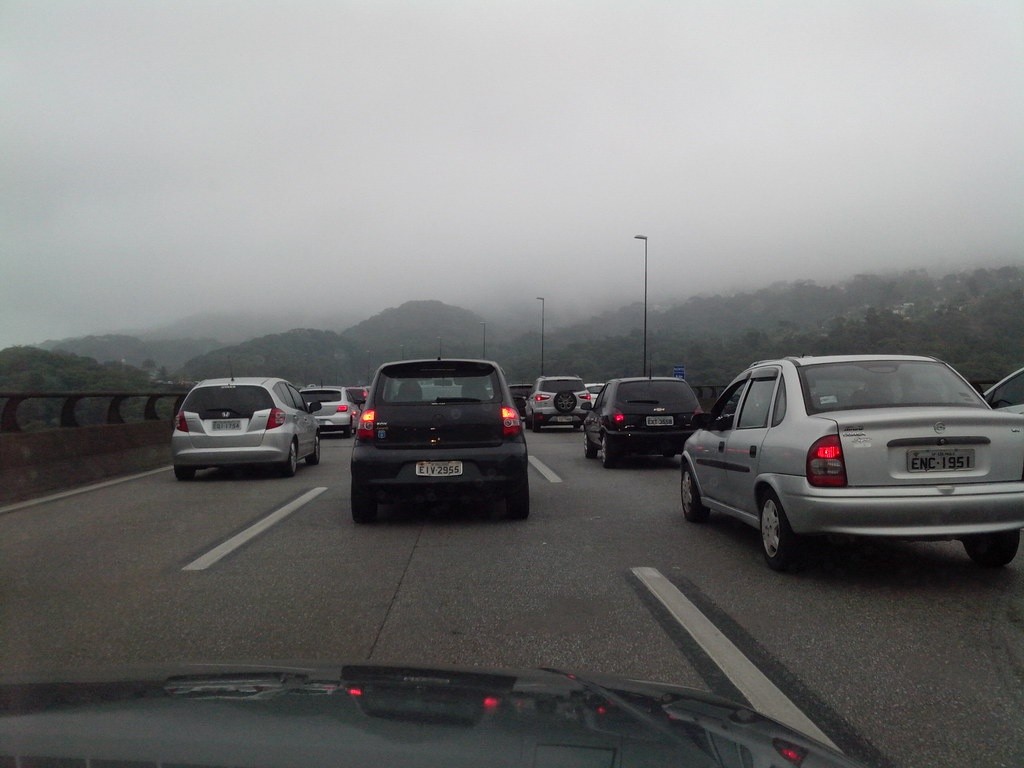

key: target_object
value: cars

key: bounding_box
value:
[584,383,606,409]
[418,385,461,400]
[347,387,369,410]
[580,377,705,467]
[680,353,1024,565]
[171,377,323,478]
[978,368,1023,416]
[351,360,529,520]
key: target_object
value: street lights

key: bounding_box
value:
[480,322,485,360]
[400,345,403,360]
[537,297,544,375]
[437,337,441,359]
[633,234,648,375]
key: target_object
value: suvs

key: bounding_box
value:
[524,376,593,432]
[508,383,532,419]
[299,384,363,436]
